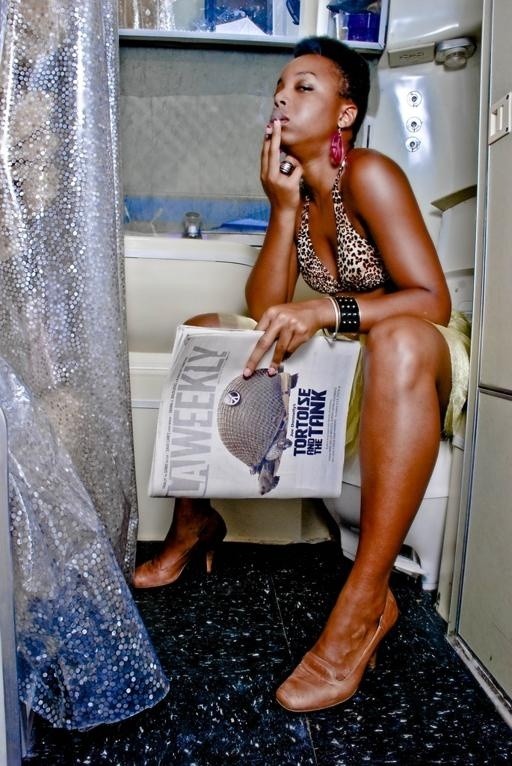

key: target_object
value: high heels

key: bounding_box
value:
[132,508,227,588]
[275,584,401,713]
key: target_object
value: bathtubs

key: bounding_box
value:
[124,234,330,545]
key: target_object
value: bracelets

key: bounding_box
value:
[322,293,360,339]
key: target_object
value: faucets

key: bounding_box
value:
[184,213,204,239]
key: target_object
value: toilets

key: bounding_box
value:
[322,198,477,591]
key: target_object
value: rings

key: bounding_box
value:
[279,161,293,175]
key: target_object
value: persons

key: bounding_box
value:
[132,38,473,714]
